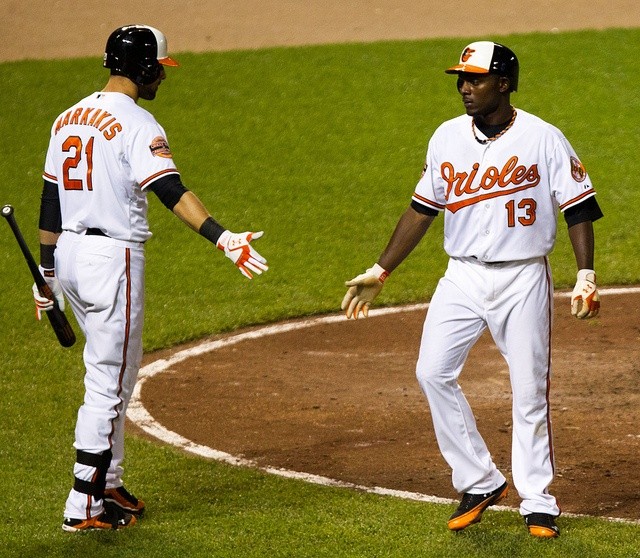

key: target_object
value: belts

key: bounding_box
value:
[62,228,111,237]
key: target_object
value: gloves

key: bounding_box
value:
[31,264,65,321]
[570,269,600,320]
[215,229,270,280]
[340,262,390,321]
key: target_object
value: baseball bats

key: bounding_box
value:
[0,206,76,347]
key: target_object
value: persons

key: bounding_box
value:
[32,21,269,536]
[340,39,604,543]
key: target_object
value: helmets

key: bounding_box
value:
[445,40,520,92]
[103,24,180,85]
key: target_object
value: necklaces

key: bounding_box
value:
[470,100,517,145]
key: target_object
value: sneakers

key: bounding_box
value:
[61,510,137,533]
[104,486,145,515]
[524,513,560,539]
[447,481,510,532]
